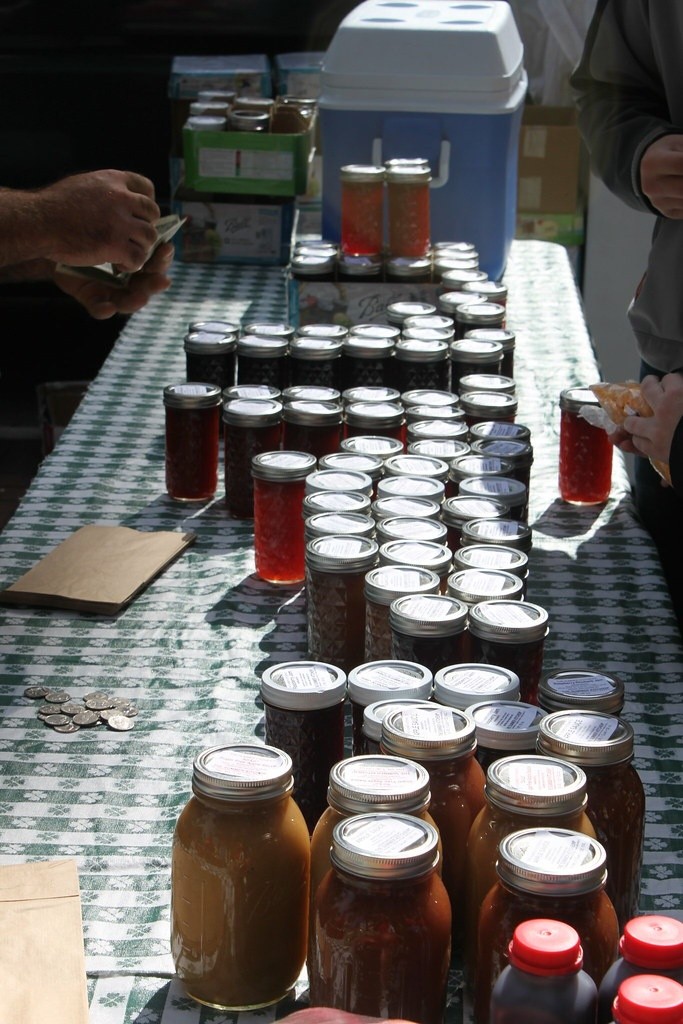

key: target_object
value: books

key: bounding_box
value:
[1,523,196,616]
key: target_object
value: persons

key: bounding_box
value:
[0,169,175,321]
[569,0,682,629]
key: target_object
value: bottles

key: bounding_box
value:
[160,90,683,1024]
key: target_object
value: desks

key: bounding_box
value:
[0,234,683,1024]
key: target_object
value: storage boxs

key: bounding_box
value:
[168,0,586,301]
